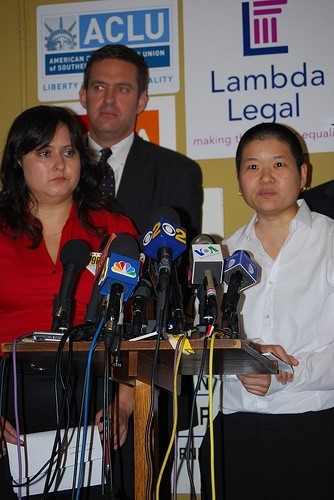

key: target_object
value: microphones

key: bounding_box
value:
[50,239,91,333]
[83,207,224,346]
[221,250,258,328]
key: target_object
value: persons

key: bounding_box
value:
[79,44,204,500]
[199,123,334,500]
[0,105,155,500]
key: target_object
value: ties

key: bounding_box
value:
[96,147,116,211]
